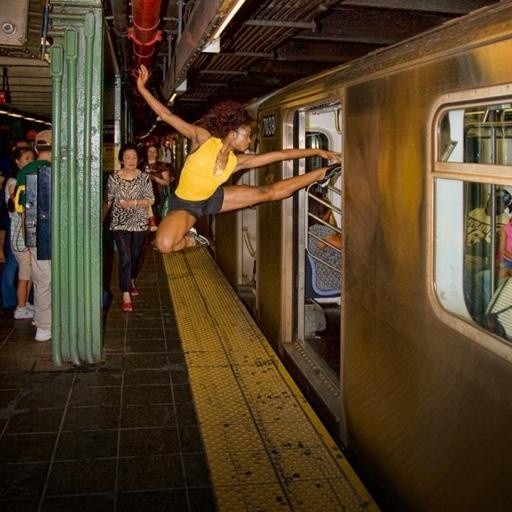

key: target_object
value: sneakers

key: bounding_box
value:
[184,226,211,248]
[12,301,35,320]
[34,326,52,343]
[316,162,343,188]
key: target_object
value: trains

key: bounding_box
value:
[161,0,512,512]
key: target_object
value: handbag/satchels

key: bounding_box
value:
[145,197,158,232]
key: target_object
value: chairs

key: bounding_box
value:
[305,254,343,306]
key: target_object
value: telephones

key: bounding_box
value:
[14,174,37,247]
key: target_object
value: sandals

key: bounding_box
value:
[121,287,140,313]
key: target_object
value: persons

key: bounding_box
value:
[137,63,344,255]
[479,271,512,343]
[473,214,511,322]
[1,129,178,342]
[467,187,511,304]
[307,191,341,292]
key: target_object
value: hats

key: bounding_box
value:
[33,128,53,148]
